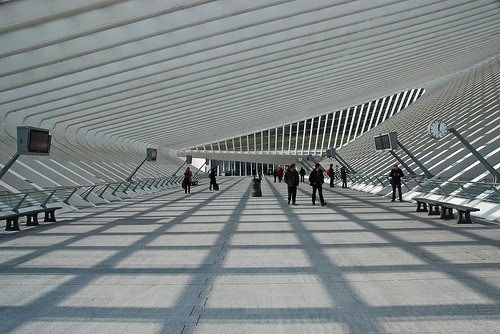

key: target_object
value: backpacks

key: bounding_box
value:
[326,168,331,175]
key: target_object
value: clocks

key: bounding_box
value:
[427,120,448,139]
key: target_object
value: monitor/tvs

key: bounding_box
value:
[326,148,335,158]
[306,154,312,162]
[206,158,210,165]
[373,132,397,152]
[17,125,50,156]
[147,148,157,161]
[187,155,192,164]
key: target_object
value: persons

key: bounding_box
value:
[210,166,217,191]
[309,164,327,207]
[300,167,305,182]
[389,165,404,202]
[273,169,277,182]
[184,167,192,194]
[329,163,335,187]
[278,167,282,182]
[341,166,349,188]
[284,163,299,205]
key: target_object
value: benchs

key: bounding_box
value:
[191,180,198,186]
[0,205,62,231]
[412,198,480,224]
